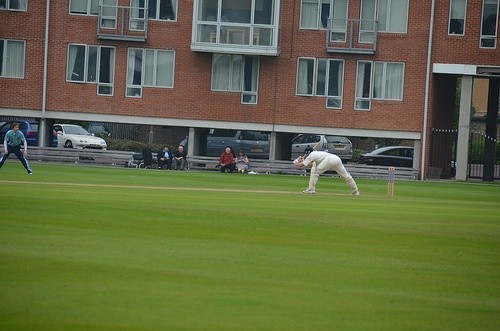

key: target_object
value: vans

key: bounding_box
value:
[177,128,270,158]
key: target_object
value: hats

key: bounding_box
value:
[304,145,313,153]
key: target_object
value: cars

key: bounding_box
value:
[52,123,107,150]
[0,120,57,148]
[288,133,352,163]
[358,145,414,168]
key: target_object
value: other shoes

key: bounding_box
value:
[167,168,171,170]
[158,166,162,169]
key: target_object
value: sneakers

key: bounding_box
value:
[28,170,33,175]
[302,188,316,194]
[352,191,359,196]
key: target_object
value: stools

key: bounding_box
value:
[253,33,259,45]
[210,32,216,43]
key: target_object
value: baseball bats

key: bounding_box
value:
[301,141,321,159]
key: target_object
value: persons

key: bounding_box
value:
[218,146,235,173]
[156,146,172,171]
[0,122,35,175]
[235,151,249,174]
[293,145,359,196]
[174,145,186,170]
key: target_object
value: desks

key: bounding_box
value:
[224,27,248,44]
